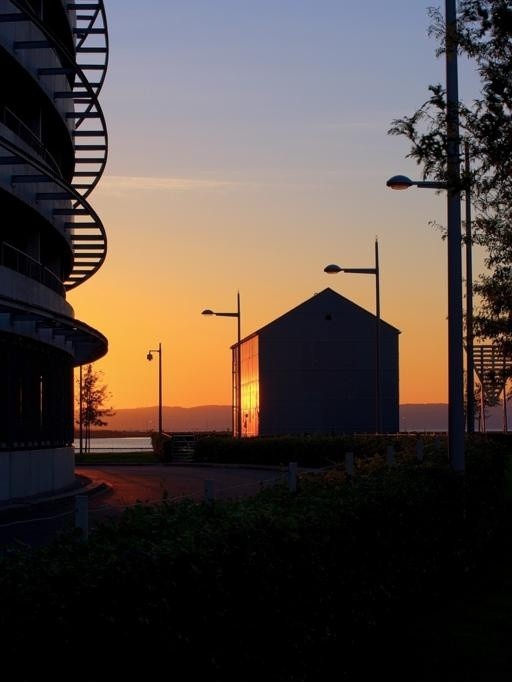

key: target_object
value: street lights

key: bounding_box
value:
[200,291,240,343]
[384,139,478,440]
[323,233,380,319]
[146,341,162,434]
